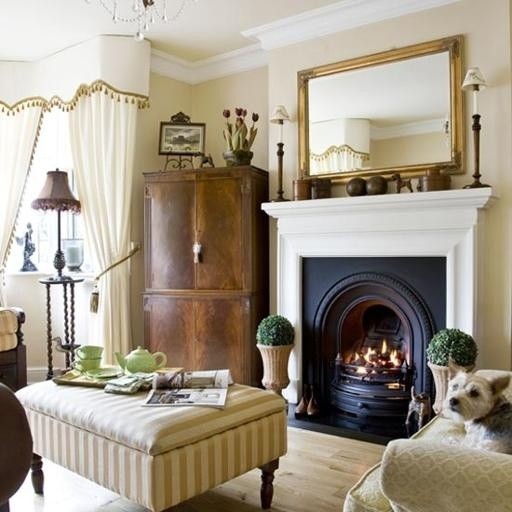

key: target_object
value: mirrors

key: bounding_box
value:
[297,33,468,186]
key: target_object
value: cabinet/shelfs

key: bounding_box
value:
[141,164,270,387]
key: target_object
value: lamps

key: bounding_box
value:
[267,105,292,203]
[82,1,194,40]
[459,67,492,189]
[31,168,83,277]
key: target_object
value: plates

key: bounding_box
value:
[85,367,122,378]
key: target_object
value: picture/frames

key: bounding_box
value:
[157,121,207,157]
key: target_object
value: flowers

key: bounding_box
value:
[220,107,260,153]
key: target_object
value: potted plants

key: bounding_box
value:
[424,328,481,418]
[257,314,295,403]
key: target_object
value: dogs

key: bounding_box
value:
[442,355,512,455]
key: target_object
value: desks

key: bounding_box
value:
[36,278,83,380]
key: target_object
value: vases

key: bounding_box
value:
[222,149,254,168]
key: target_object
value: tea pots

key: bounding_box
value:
[113,346,168,374]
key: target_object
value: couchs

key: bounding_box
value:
[339,369,509,512]
[0,381,33,512]
[0,305,27,391]
[14,370,287,512]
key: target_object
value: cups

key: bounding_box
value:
[76,346,104,359]
[73,360,101,373]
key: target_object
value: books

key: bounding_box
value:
[141,368,234,410]
